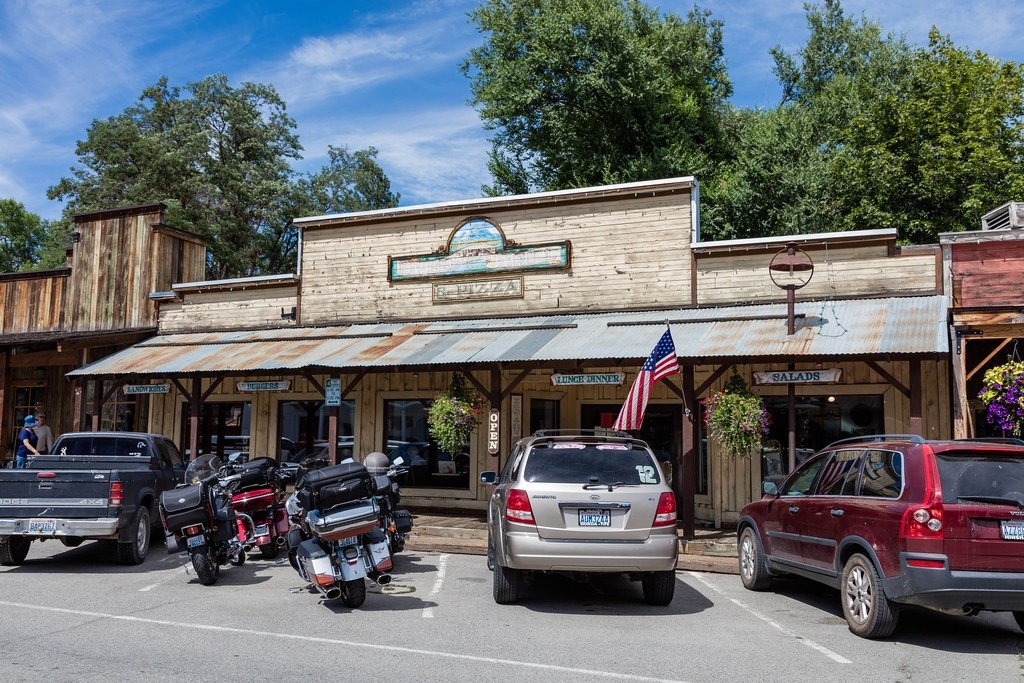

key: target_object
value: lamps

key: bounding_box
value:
[769,243,814,276]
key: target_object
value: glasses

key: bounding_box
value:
[38,416,47,419]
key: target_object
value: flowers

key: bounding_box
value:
[423,369,490,462]
[977,361,1023,438]
[700,363,774,464]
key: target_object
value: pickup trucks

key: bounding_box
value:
[0,430,191,571]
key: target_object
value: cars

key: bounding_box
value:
[210,435,470,476]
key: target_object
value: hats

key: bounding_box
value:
[24,415,39,427]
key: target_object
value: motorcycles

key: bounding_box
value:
[159,452,414,608]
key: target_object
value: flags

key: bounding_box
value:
[610,329,681,433]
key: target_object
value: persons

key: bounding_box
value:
[31,411,53,456]
[16,414,41,470]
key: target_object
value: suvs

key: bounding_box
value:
[735,433,1024,640]
[479,428,679,606]
